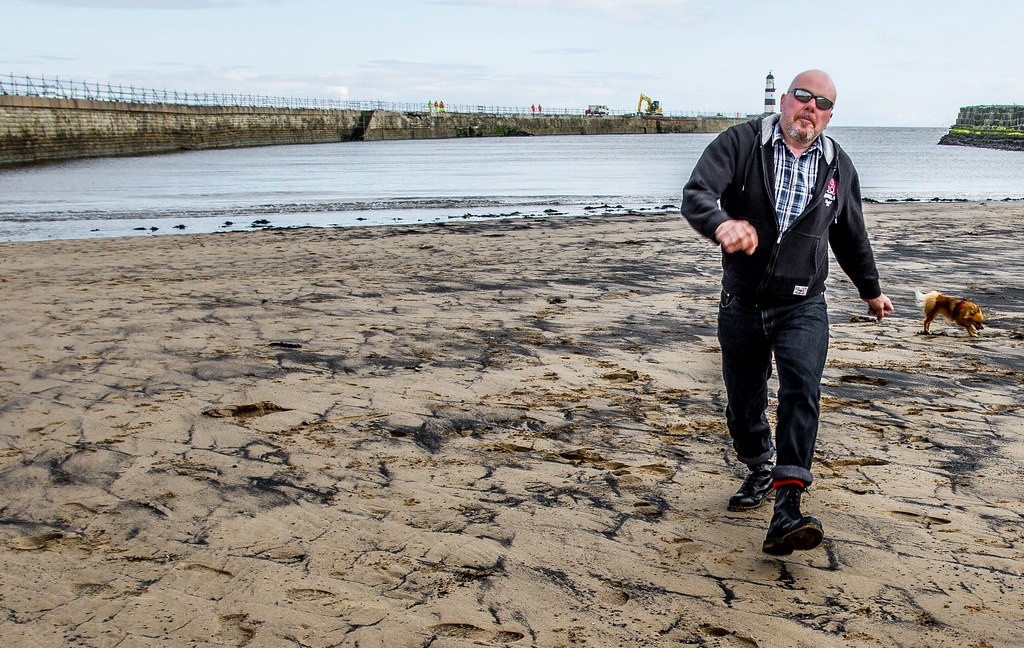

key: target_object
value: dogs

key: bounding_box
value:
[913,288,985,337]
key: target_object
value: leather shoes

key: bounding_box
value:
[727,460,776,511]
[762,484,824,555]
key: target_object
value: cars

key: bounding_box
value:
[585,105,610,115]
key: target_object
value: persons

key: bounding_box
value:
[428,100,445,112]
[680,69,893,556]
[532,104,541,112]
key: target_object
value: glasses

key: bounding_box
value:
[788,88,834,110]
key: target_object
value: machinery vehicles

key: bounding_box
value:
[637,94,664,117]
